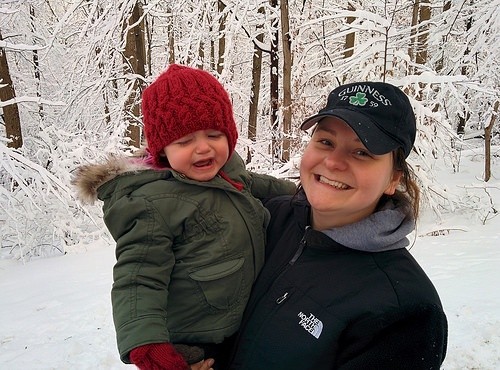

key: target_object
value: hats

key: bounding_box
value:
[140,64,238,160]
[300,82,417,157]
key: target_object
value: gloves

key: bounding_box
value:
[130,343,188,370]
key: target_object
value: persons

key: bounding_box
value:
[77,63,298,370]
[189,82,448,370]
